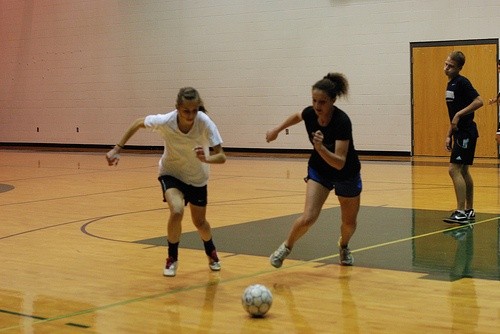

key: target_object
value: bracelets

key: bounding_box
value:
[117,143,124,148]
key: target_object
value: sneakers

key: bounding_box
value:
[269,240,291,268]
[163,257,178,276]
[337,236,354,266]
[443,210,469,224]
[444,224,475,240]
[464,209,475,223]
[207,249,221,271]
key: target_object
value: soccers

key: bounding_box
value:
[241,284,273,316]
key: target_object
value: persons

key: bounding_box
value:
[105,87,226,277]
[443,51,500,224]
[266,72,362,269]
